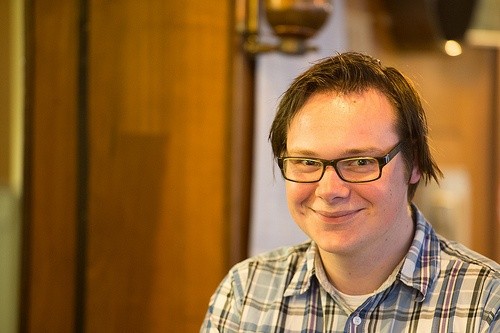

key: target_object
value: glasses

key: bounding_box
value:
[277,141,404,184]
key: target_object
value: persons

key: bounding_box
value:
[199,51,500,333]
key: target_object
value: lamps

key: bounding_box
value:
[233,0,335,55]
